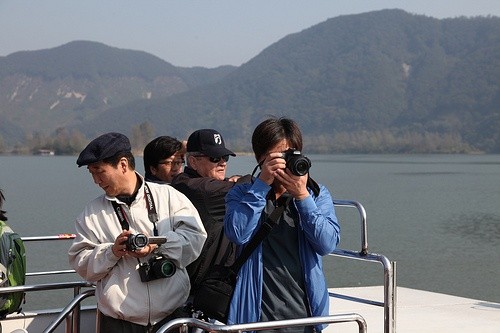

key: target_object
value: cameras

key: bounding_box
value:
[124,233,148,251]
[137,256,176,282]
[273,148,312,176]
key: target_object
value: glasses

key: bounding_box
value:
[159,161,185,166]
[193,154,229,163]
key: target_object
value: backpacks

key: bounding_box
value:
[0,221,26,316]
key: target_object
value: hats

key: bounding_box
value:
[187,129,236,157]
[75,132,131,168]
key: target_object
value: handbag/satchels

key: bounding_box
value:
[193,264,237,313]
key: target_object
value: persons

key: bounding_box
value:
[143,137,187,187]
[68,132,207,333]
[224,113,340,333]
[171,129,256,324]
